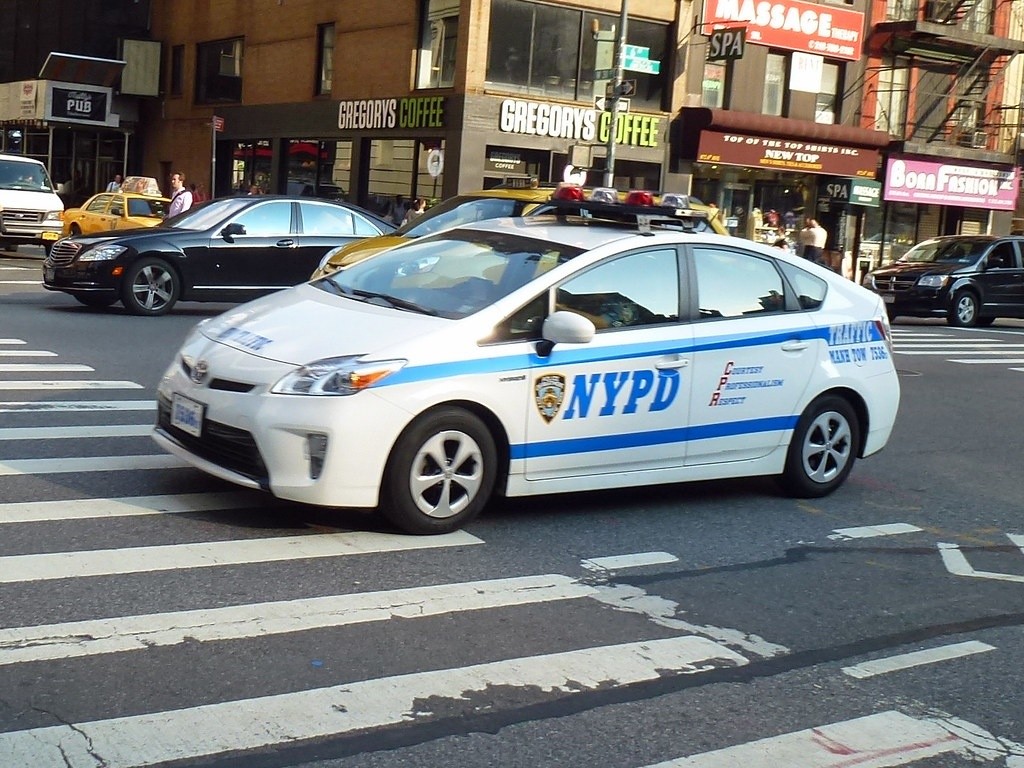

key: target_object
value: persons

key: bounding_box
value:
[107,175,122,193]
[165,170,193,220]
[773,218,827,264]
[387,195,427,226]
[557,293,638,328]
[190,184,200,201]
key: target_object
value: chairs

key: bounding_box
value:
[542,76,593,97]
[988,248,1014,268]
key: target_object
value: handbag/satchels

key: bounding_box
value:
[400,210,411,228]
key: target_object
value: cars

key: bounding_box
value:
[145,185,903,540]
[866,232,898,242]
[862,233,1024,327]
[40,192,449,318]
[311,185,734,328]
[61,175,173,238]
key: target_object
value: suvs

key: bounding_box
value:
[0,153,66,258]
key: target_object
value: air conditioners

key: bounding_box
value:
[925,0,957,25]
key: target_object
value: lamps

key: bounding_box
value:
[961,130,988,148]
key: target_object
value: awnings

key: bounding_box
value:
[679,106,889,179]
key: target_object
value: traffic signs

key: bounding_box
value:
[605,80,637,98]
[595,97,630,113]
